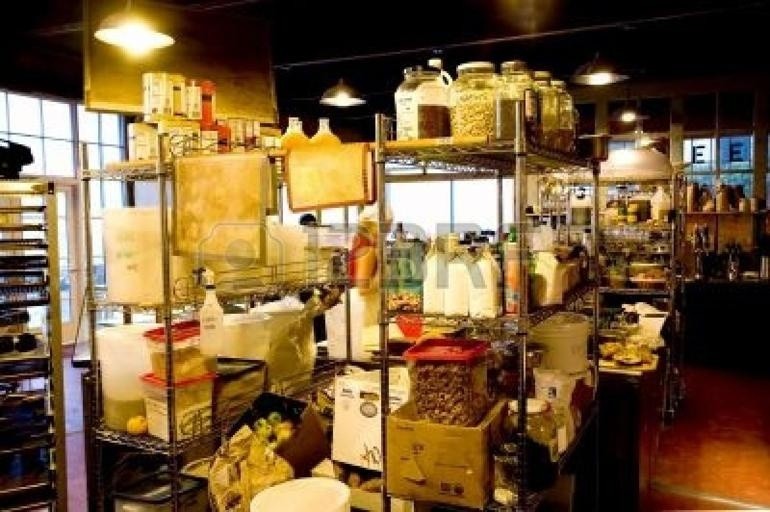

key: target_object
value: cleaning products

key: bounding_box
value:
[190,265,224,356]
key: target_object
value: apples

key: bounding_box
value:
[254,411,291,448]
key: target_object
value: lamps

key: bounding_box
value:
[319,76,367,107]
[567,48,630,86]
[608,100,650,124]
[577,132,611,363]
[94,1,176,49]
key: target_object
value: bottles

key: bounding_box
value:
[282,117,342,146]
[546,387,567,457]
[422,235,503,319]
[738,193,758,212]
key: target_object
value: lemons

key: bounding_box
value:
[127,415,148,436]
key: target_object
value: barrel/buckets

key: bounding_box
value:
[423,229,503,320]
[528,310,590,373]
[250,477,352,512]
[651,186,671,219]
[94,322,167,432]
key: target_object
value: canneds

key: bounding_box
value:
[394,60,575,153]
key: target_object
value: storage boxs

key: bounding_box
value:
[225,391,330,480]
[384,395,513,512]
[141,359,267,440]
[403,337,493,425]
[329,367,421,473]
[140,319,209,382]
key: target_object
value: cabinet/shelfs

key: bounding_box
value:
[566,312,675,512]
[1,179,67,512]
[534,165,685,420]
[78,100,351,512]
[367,103,604,512]
